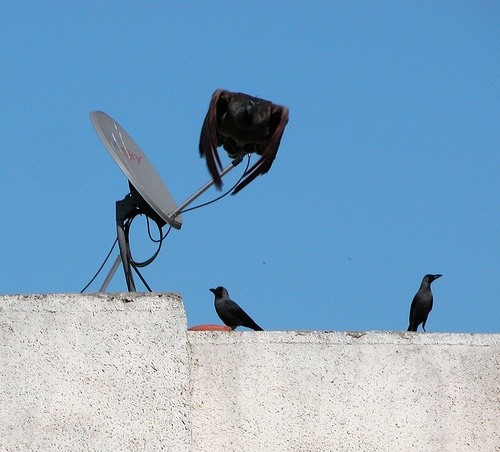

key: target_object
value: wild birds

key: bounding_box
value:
[199,87,290,194]
[209,286,264,331]
[407,274,443,331]
